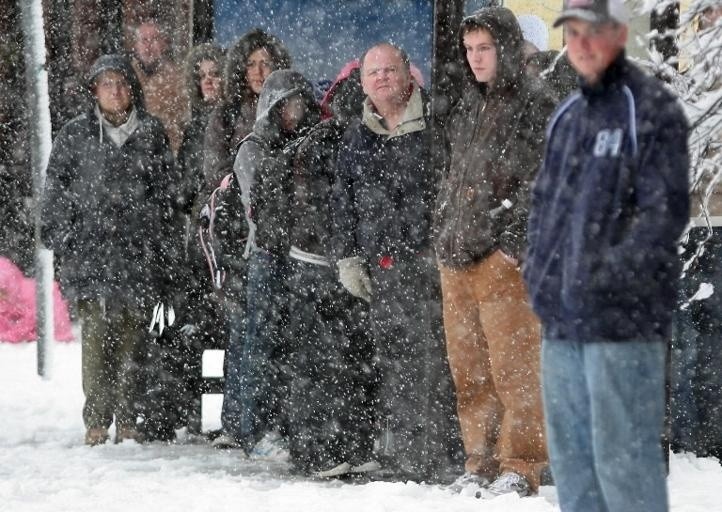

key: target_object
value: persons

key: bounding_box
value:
[521,1,691,458]
[174,42,227,205]
[0,256,74,344]
[432,7,560,500]
[126,19,192,150]
[233,70,322,464]
[39,53,202,446]
[333,43,467,485]
[204,29,290,190]
[282,69,382,482]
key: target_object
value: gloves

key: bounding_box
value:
[337,257,371,302]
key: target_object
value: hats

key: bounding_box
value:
[552,0,627,29]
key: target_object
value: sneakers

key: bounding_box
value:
[211,434,240,448]
[350,454,381,472]
[248,431,291,462]
[444,471,489,498]
[307,451,350,478]
[84,425,110,445]
[484,471,531,499]
[114,423,141,444]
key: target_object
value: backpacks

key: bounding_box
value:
[198,171,249,297]
